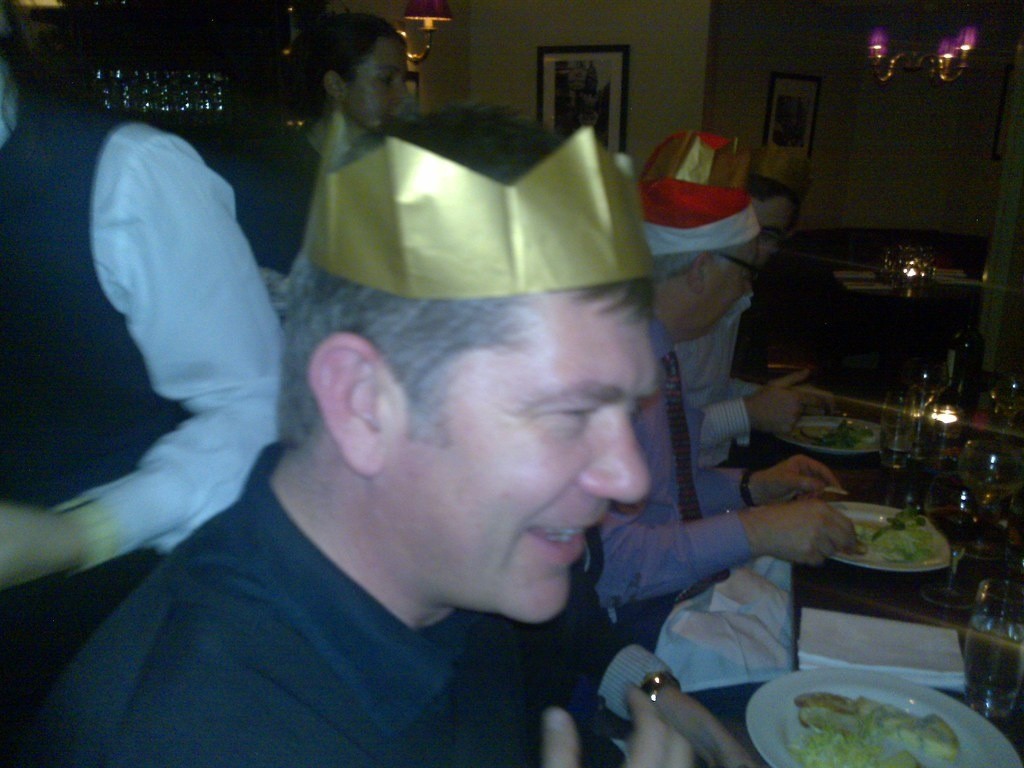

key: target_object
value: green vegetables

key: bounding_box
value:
[873,502,928,539]
[800,419,875,451]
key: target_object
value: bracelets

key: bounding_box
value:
[741,464,768,507]
[638,672,681,700]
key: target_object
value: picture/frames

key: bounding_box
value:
[762,72,823,158]
[535,44,630,153]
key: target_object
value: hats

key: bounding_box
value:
[635,126,761,253]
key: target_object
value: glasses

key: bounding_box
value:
[719,251,761,282]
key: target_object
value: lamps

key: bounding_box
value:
[857,0,978,90]
[392,0,453,64]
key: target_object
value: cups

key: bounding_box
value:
[881,374,961,468]
[963,579,1024,717]
[87,66,232,111]
[884,244,936,289]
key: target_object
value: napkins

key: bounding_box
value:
[799,604,965,692]
[672,569,796,668]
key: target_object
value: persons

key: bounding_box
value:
[33,106,750,768]
[238,12,410,281]
[0,59,285,726]
[593,129,867,626]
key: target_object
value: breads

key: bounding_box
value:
[795,691,959,768]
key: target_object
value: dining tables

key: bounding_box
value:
[608,227,1024,768]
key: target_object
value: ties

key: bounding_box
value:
[664,351,731,584]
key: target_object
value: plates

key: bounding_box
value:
[819,500,952,570]
[745,668,1022,768]
[773,415,885,453]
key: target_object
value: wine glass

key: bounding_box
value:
[991,376,1024,441]
[919,441,1024,607]
[919,357,949,405]
